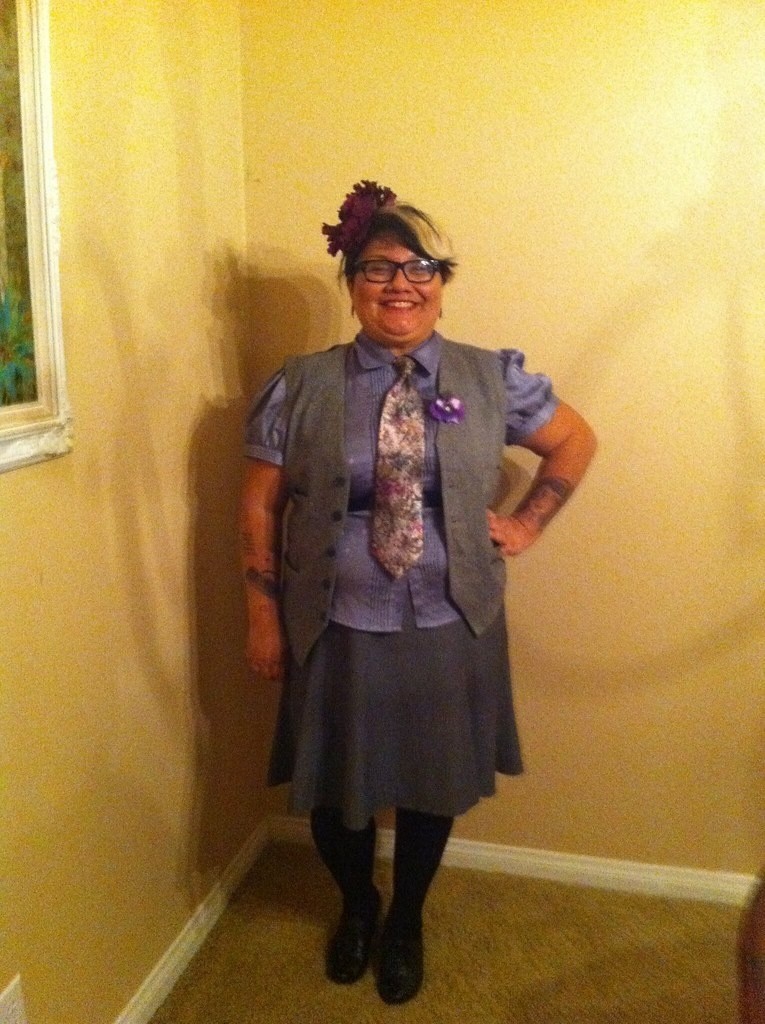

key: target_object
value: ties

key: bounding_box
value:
[370,355,426,581]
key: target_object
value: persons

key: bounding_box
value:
[235,178,598,1008]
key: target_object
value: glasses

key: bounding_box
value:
[350,259,441,283]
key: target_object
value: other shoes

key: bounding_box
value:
[325,892,381,985]
[373,910,424,1006]
